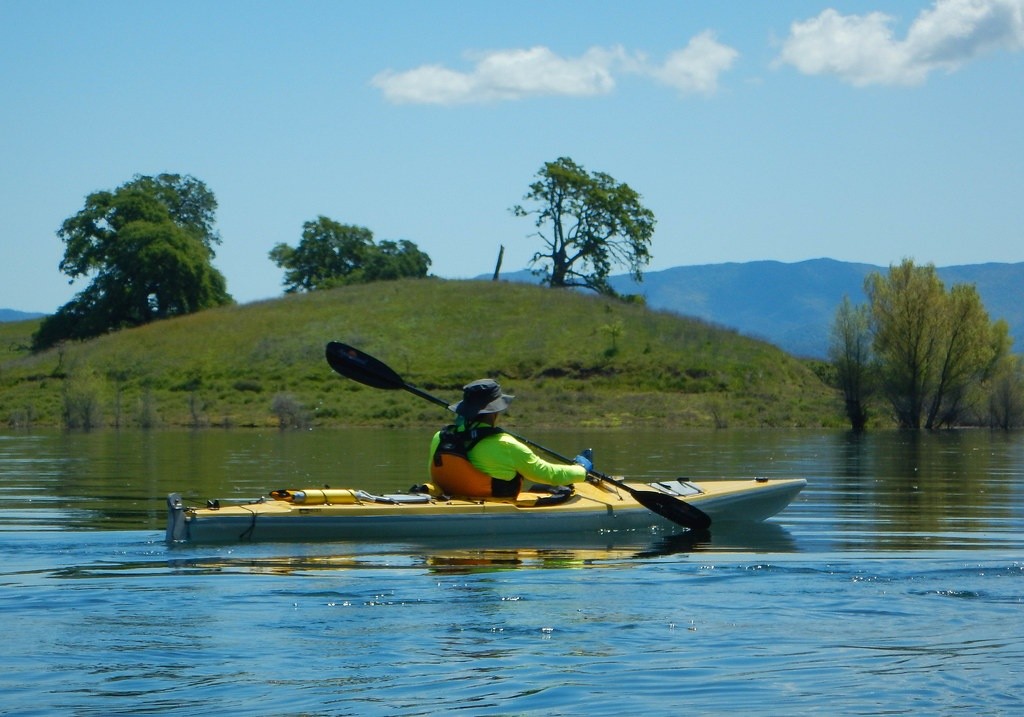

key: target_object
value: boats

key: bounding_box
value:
[166,469,810,546]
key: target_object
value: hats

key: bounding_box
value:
[448,379,515,416]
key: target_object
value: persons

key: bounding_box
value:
[429,378,593,498]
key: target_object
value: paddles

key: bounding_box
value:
[325,336,710,531]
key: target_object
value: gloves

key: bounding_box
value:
[573,454,593,475]
